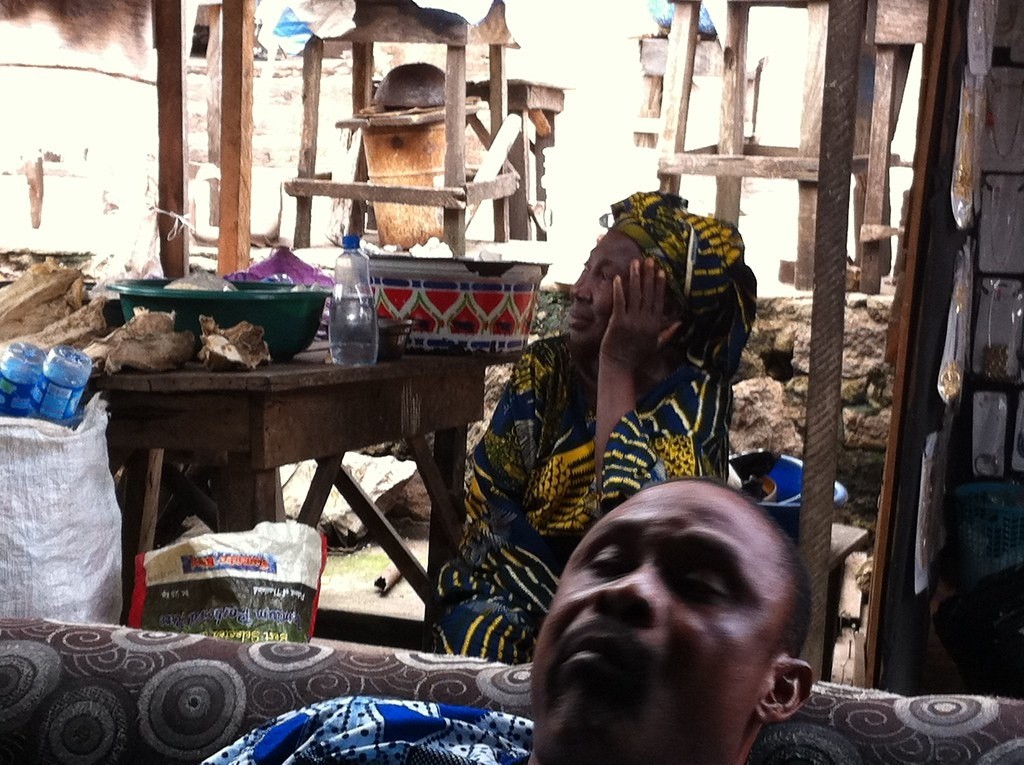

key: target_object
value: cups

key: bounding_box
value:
[328,234,379,367]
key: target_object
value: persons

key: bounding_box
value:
[196,478,815,764]
[433,191,757,665]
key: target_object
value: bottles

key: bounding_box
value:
[28,345,93,425]
[0,342,46,419]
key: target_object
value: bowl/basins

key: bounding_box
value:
[104,278,333,364]
[724,451,851,545]
[320,318,416,360]
[756,475,778,502]
[368,253,553,358]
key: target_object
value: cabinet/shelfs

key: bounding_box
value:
[282,1,570,299]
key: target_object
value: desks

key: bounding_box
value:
[84,336,527,661]
